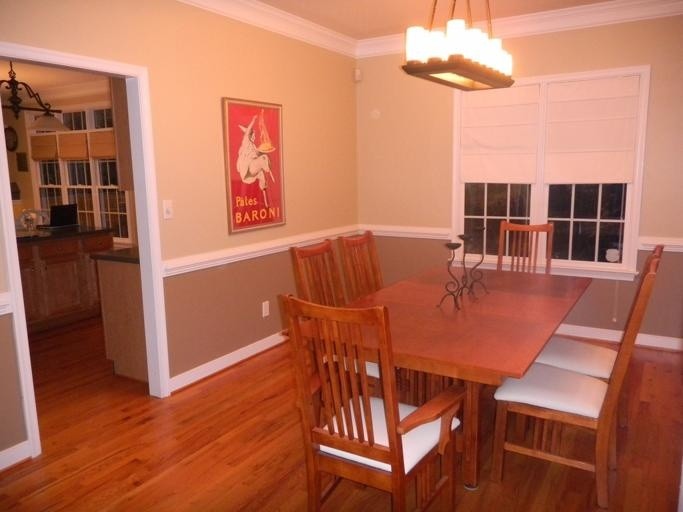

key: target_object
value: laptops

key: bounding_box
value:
[36,204,77,229]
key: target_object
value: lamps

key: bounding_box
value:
[0,60,71,131]
[402,0,515,91]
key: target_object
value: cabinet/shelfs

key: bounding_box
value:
[16,227,115,334]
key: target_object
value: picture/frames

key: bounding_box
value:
[223,96,286,235]
